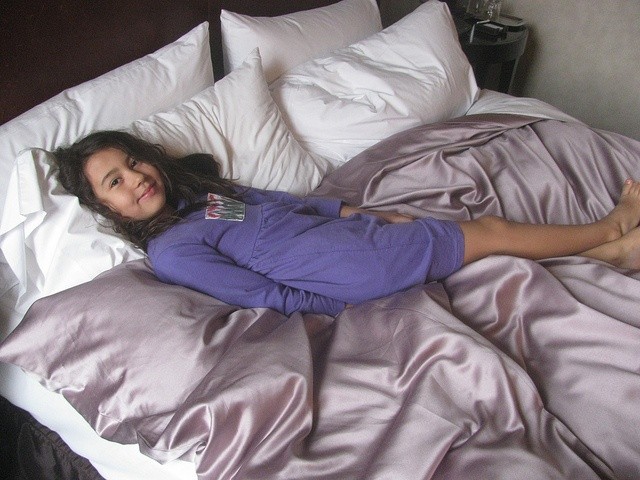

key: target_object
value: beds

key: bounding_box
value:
[1,0,639,478]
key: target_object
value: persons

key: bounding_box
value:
[54,131,640,318]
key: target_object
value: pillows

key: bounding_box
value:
[0,45,330,318]
[219,0,384,86]
[266,0,483,177]
[0,19,215,217]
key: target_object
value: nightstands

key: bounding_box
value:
[389,6,529,95]
